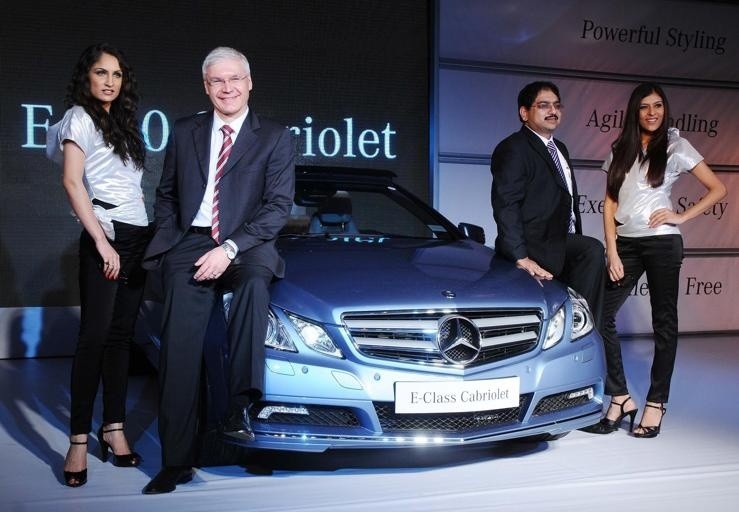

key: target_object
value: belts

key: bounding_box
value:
[188,225,209,235]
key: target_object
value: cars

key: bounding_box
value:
[129,166,613,472]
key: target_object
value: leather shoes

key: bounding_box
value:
[579,422,611,434]
[218,402,256,442]
[142,466,192,494]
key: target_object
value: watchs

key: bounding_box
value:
[221,243,236,260]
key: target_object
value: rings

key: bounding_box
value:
[104,262,109,265]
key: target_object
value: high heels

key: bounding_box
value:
[97,422,141,467]
[64,439,88,487]
[634,402,666,438]
[601,397,638,432]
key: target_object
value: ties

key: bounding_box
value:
[548,141,572,234]
[210,124,234,245]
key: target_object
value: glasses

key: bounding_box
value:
[98,263,130,284]
[530,102,564,111]
[205,72,247,88]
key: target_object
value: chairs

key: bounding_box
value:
[306,198,360,234]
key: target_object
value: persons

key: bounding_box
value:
[46,41,149,488]
[141,47,295,495]
[592,82,727,438]
[490,81,606,332]
